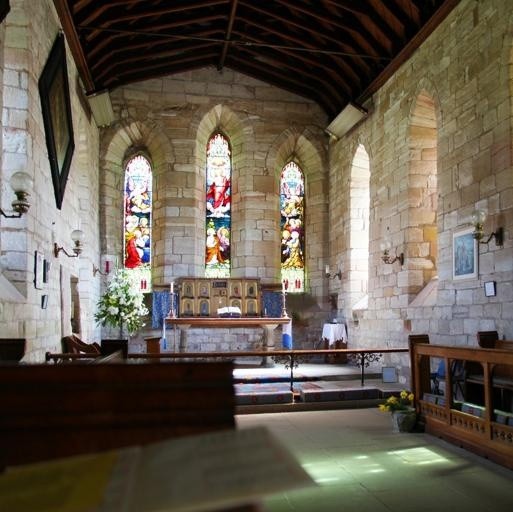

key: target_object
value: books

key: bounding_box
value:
[217,306,242,316]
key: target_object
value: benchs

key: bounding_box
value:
[463,373,513,410]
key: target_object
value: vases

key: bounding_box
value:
[390,411,416,432]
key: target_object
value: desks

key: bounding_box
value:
[165,317,291,366]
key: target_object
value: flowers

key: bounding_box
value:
[93,271,150,336]
[378,389,415,412]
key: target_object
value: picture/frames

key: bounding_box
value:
[36,32,75,209]
[451,227,478,281]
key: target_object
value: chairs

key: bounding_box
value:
[429,359,465,401]
[61,334,100,360]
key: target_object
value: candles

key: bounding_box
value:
[169,282,173,293]
[281,282,285,295]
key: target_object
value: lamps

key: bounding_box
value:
[470,209,504,246]
[0,171,34,219]
[54,229,85,258]
